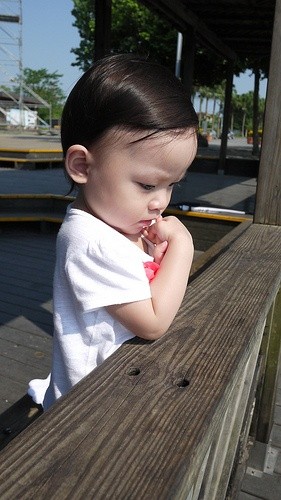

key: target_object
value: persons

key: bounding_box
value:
[21,46,203,418]
[198,121,265,147]
